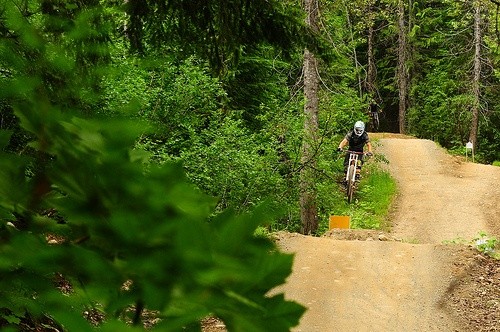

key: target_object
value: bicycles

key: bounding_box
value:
[336,148,371,204]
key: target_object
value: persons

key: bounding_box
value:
[338,121,373,191]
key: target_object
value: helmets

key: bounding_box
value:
[354,120,365,136]
